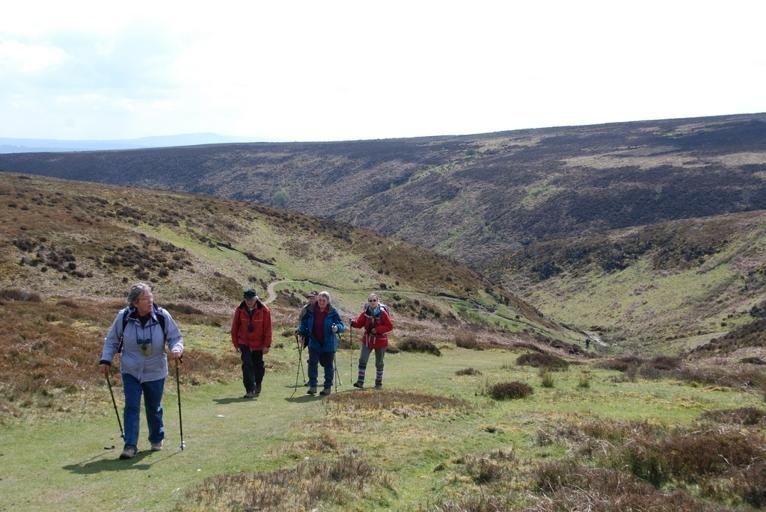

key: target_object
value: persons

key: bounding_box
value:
[348,293,393,388]
[298,291,319,387]
[586,338,590,347]
[230,288,272,399]
[295,291,344,395]
[99,283,184,459]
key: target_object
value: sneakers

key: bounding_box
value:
[120,446,137,458]
[374,384,382,389]
[151,439,162,451]
[243,391,261,398]
[354,381,363,388]
[320,388,331,396]
[305,379,317,386]
[307,387,318,394]
